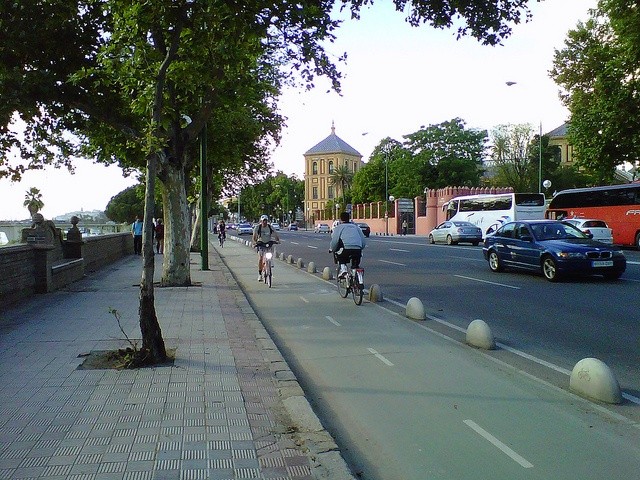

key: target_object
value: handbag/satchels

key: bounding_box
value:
[155,233,162,240]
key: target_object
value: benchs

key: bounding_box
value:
[49,257,88,290]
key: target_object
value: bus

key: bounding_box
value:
[441,193,546,241]
[545,183,640,248]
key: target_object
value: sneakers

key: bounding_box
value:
[258,275,262,281]
[338,268,348,279]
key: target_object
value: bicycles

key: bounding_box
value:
[329,250,364,306]
[217,231,227,248]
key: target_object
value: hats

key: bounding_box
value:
[261,215,268,220]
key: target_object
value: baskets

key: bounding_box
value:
[334,252,350,264]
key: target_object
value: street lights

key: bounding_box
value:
[505,82,543,194]
[275,184,288,213]
[361,131,389,235]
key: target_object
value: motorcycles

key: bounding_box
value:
[254,241,278,287]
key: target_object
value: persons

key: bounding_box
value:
[402,220,408,236]
[328,211,366,279]
[216,220,225,246]
[131,215,143,255]
[155,218,165,254]
[251,214,280,281]
[214,226,218,234]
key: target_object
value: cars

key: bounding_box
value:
[482,220,626,281]
[314,224,330,234]
[287,224,297,231]
[355,223,370,237]
[252,223,258,227]
[226,223,237,230]
[428,221,482,246]
[271,223,280,230]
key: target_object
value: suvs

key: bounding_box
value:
[561,218,614,247]
[237,224,253,236]
[331,220,353,233]
